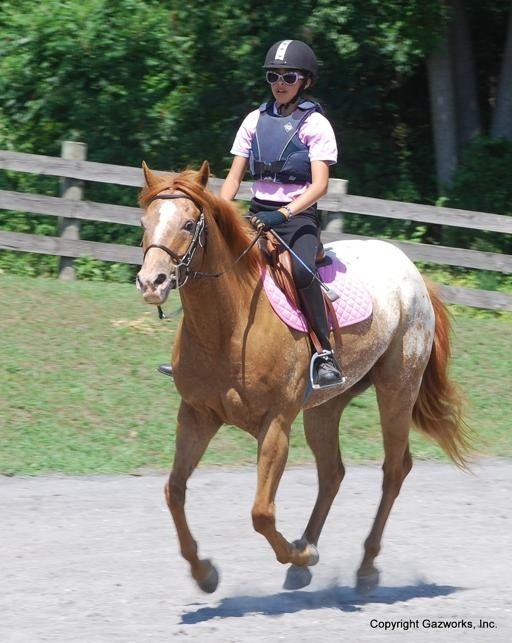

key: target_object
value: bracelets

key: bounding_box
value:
[281,205,292,224]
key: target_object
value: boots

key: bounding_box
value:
[297,274,342,386]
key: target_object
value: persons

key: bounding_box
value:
[154,38,351,394]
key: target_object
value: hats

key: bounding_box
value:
[261,39,319,80]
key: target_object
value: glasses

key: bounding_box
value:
[265,71,304,85]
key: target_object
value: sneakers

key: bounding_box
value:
[158,363,172,377]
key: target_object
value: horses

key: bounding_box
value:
[135,159,479,596]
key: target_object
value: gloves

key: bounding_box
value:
[249,210,287,231]
